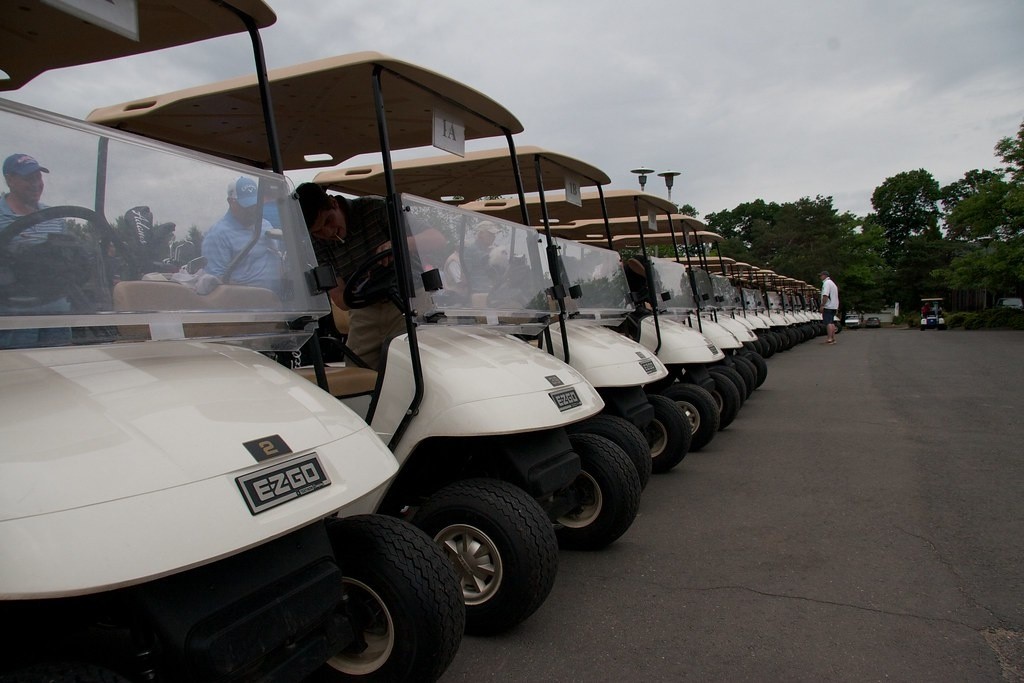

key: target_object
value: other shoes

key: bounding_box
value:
[825,339,836,345]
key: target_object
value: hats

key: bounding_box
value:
[227,176,257,208]
[473,221,502,234]
[2,154,50,177]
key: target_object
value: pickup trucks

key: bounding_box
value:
[993,297,1024,314]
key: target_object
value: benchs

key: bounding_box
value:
[476,307,539,348]
[114,282,380,396]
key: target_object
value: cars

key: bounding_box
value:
[865,316,881,328]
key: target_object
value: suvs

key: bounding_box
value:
[845,314,861,330]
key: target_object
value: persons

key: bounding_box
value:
[444,221,509,292]
[922,303,942,317]
[201,175,284,293]
[817,271,839,344]
[0,153,74,346]
[623,253,653,311]
[258,178,281,229]
[294,183,445,366]
